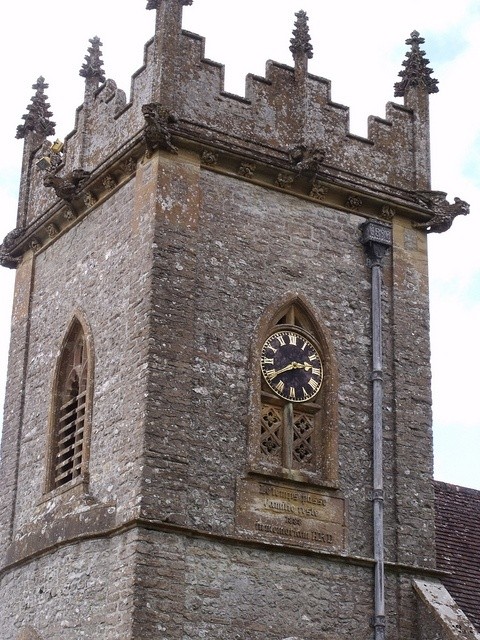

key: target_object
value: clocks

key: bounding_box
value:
[260,331,324,403]
